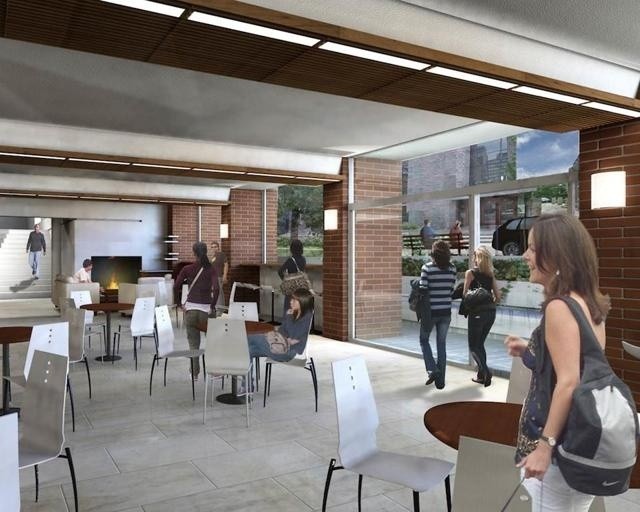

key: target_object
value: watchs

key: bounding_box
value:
[540,433,558,448]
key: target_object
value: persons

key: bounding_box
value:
[504,215,612,511]
[26,224,47,280]
[73,259,92,283]
[210,242,228,317]
[462,246,500,387]
[450,219,465,249]
[278,239,306,315]
[237,287,315,394]
[416,241,457,390]
[172,243,220,380]
[420,219,434,249]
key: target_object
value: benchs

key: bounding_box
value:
[403,232,470,255]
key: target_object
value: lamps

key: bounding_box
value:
[324,209,338,231]
[590,171,625,210]
[220,224,229,239]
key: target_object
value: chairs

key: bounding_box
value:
[322,356,455,512]
[507,356,533,404]
[453,434,534,512]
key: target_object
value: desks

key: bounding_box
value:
[423,401,523,450]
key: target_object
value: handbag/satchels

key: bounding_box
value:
[279,269,311,296]
[462,286,496,316]
[266,330,290,356]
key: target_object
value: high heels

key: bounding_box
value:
[484,372,493,388]
[472,375,487,383]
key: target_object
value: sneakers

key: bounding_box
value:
[189,373,199,382]
[236,386,255,397]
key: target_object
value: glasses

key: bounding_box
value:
[211,246,218,248]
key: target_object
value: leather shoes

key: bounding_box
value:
[435,372,446,390]
[425,368,441,385]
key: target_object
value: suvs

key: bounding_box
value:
[492,216,538,256]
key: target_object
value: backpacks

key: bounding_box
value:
[535,295,640,496]
